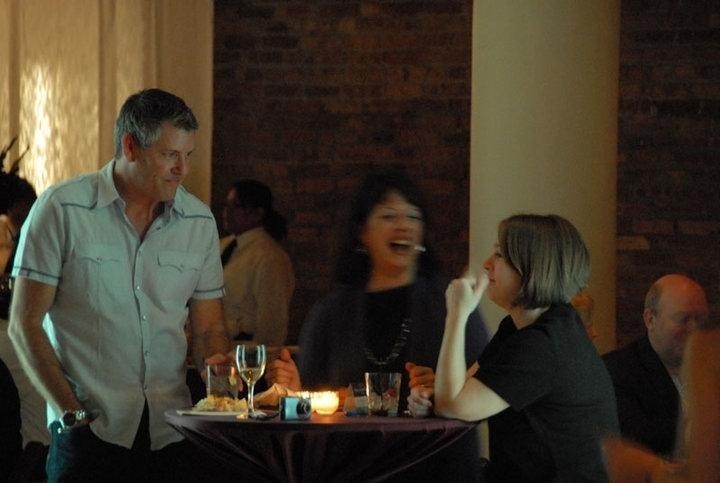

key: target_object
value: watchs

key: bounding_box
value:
[59,408,86,430]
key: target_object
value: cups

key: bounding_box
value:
[204,363,240,400]
[364,372,402,418]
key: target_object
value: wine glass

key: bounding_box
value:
[236,343,268,420]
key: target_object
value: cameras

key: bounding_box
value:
[279,396,313,421]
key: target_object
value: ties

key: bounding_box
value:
[220,240,236,265]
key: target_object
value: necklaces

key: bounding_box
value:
[362,317,411,366]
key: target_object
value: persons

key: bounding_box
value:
[0,171,37,276]
[406,213,623,483]
[6,87,231,483]
[218,179,295,345]
[264,176,489,411]
[598,273,710,462]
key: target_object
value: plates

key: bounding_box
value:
[177,409,244,416]
